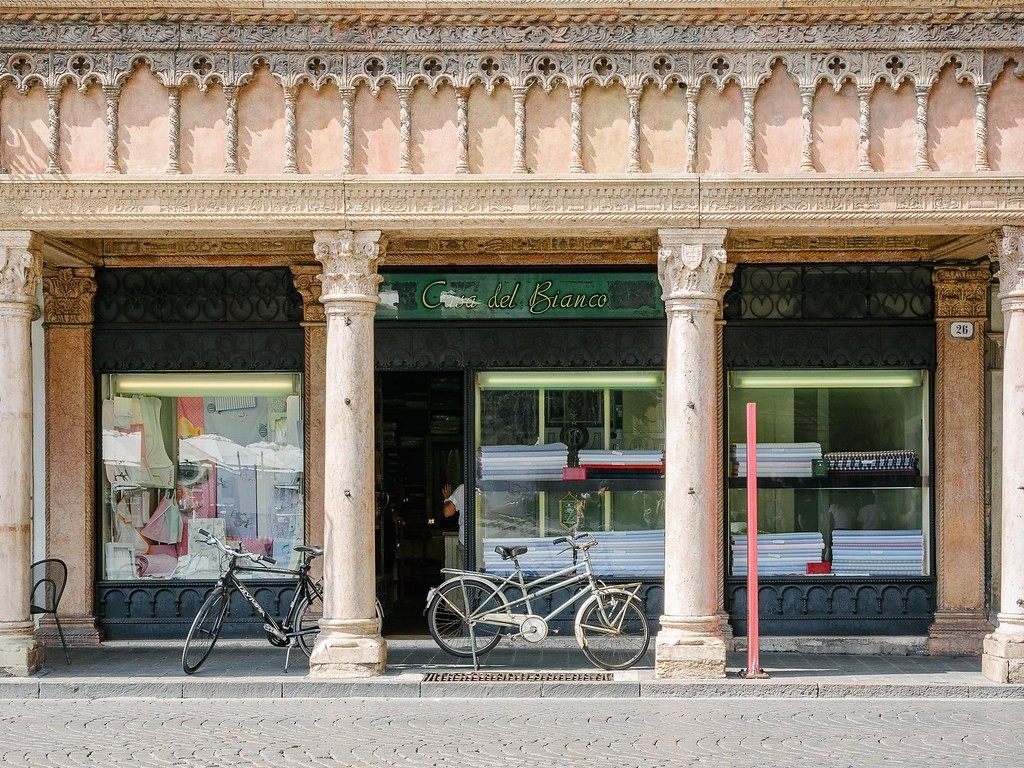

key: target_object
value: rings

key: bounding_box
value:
[445,490,448,494]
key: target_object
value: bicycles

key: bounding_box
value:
[182,529,385,675]
[423,532,651,672]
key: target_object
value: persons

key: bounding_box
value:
[441,431,464,577]
[827,489,922,531]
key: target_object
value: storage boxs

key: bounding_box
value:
[188,519,226,553]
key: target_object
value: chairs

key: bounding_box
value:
[31,558,71,665]
